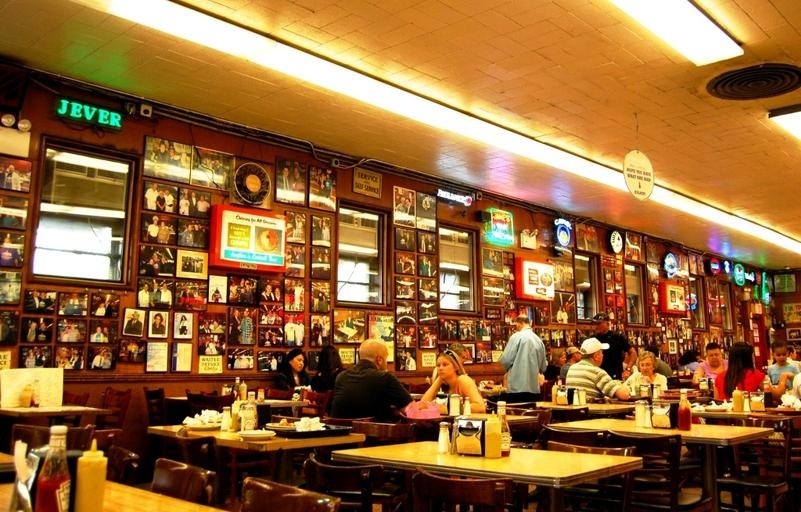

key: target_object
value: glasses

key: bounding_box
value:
[436,349,457,361]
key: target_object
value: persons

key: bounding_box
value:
[559,340,676,399]
[497,310,549,405]
[1,137,801,373]
[585,311,636,384]
[414,349,490,443]
[680,336,801,407]
[317,338,418,511]
[263,346,313,399]
[302,345,346,401]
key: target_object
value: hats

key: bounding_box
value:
[590,312,611,323]
[579,337,610,355]
[566,346,579,356]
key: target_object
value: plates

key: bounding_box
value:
[478,381,506,393]
[185,412,297,440]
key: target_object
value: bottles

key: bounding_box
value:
[33,424,72,511]
[630,383,693,431]
[703,389,801,415]
[552,382,588,406]
[219,376,266,433]
[21,379,41,408]
[673,365,715,397]
[435,391,512,459]
[74,438,108,512]
